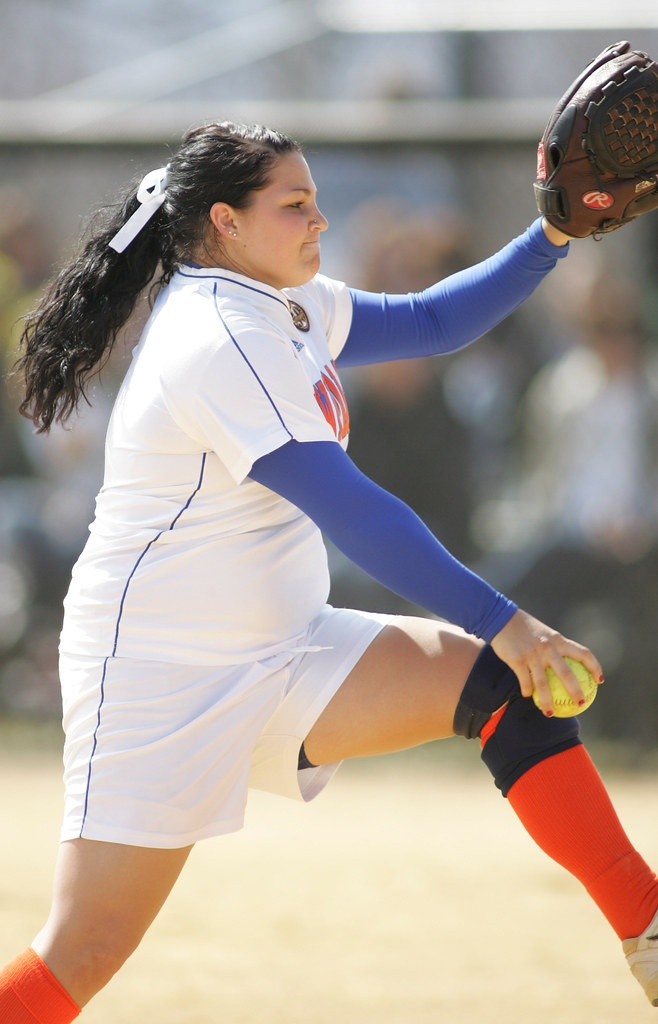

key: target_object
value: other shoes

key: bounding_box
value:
[620,906,658,1007]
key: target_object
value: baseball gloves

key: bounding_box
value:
[535,39,658,242]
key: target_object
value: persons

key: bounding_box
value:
[0,125,657,1024]
[0,193,658,751]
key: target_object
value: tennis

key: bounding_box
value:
[527,647,599,719]
